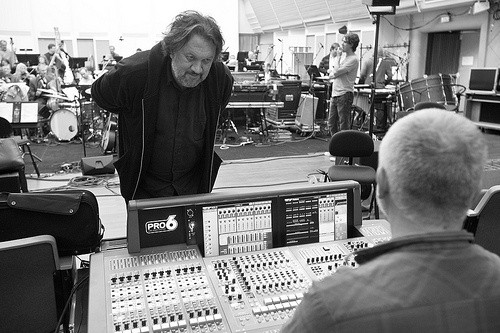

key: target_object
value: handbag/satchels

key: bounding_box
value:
[0,139,25,172]
[0,188,105,257]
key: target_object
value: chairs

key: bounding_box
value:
[324,130,380,219]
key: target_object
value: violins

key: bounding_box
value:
[22,73,35,79]
[9,37,17,69]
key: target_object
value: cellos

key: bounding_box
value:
[55,40,74,85]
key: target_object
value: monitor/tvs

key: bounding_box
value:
[466,67,499,95]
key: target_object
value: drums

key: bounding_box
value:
[48,109,78,141]
[395,74,459,112]
[81,102,102,125]
[59,102,80,116]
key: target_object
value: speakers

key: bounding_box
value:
[82,155,115,175]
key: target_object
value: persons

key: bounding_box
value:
[92,10,234,211]
[242,52,257,71]
[358,47,392,88]
[281,107,500,333]
[136,48,141,52]
[105,46,118,56]
[328,33,360,164]
[318,43,340,76]
[0,40,70,134]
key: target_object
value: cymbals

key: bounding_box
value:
[85,88,91,95]
[42,93,72,102]
[36,88,65,96]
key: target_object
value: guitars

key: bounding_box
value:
[100,112,116,151]
